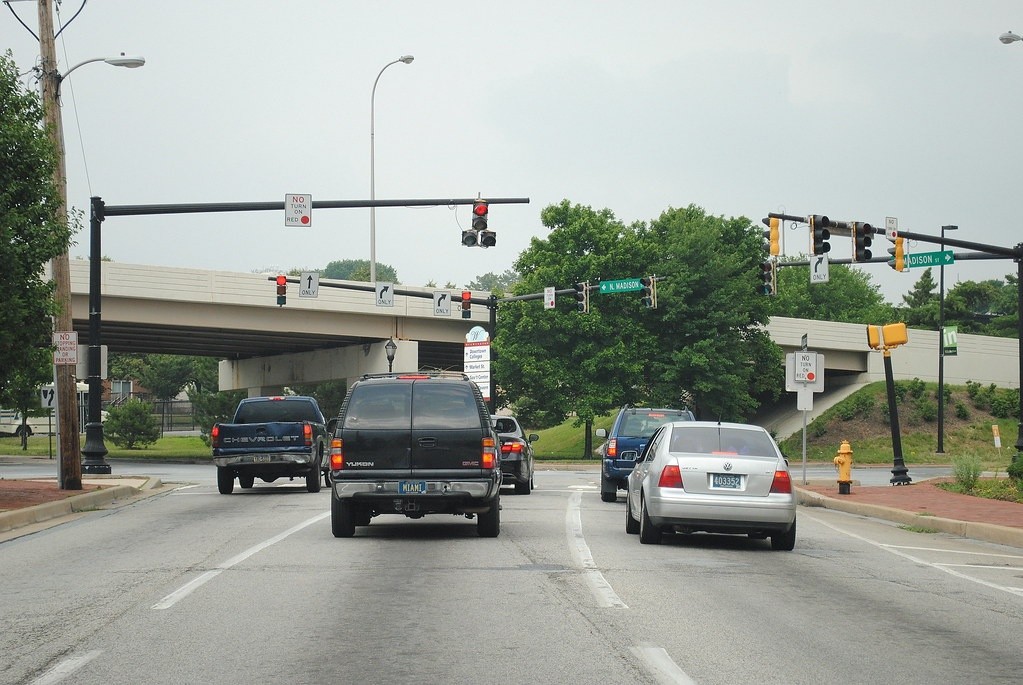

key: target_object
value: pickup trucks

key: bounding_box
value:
[211,395,338,495]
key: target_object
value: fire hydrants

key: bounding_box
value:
[833,440,856,495]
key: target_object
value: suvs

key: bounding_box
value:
[328,370,516,539]
[596,403,697,504]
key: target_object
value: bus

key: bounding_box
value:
[0,382,112,436]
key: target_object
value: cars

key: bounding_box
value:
[488,414,540,495]
[619,420,798,550]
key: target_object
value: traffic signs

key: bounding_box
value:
[888,250,955,269]
[298,272,318,297]
[599,277,645,294]
[432,290,452,316]
[375,281,394,308]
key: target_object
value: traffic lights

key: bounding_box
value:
[574,281,590,316]
[850,219,874,263]
[887,236,906,272]
[462,231,496,249]
[276,276,287,306]
[808,254,831,286]
[462,290,474,318]
[473,200,488,231]
[809,213,833,256]
[867,324,881,349]
[640,275,657,309]
[762,217,782,256]
[760,260,778,298]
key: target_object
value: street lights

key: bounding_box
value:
[36,52,147,492]
[938,225,958,450]
[368,54,417,286]
[385,336,399,378]
